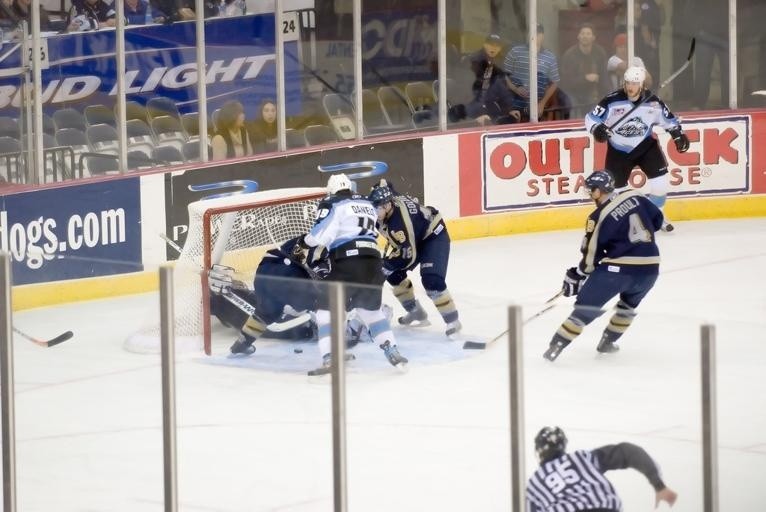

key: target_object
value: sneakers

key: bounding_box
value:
[597,331,619,352]
[542,338,567,361]
[660,220,673,231]
[229,300,460,376]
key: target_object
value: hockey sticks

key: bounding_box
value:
[12,328,73,347]
[464,288,566,348]
[607,38,696,130]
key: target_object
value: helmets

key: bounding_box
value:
[584,171,614,193]
[327,173,351,194]
[367,185,392,205]
[535,426,566,461]
[623,67,645,82]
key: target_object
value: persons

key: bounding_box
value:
[585,1,664,54]
[230,231,365,356]
[584,65,689,234]
[519,426,678,512]
[369,180,463,336]
[10,1,227,31]
[605,33,652,106]
[211,101,253,163]
[563,22,607,117]
[255,101,282,144]
[545,169,665,361]
[294,172,409,368]
[502,24,562,120]
[453,32,521,122]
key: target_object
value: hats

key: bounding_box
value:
[485,23,627,47]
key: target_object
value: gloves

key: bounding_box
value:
[670,129,688,153]
[291,234,311,264]
[562,267,586,296]
[592,124,613,142]
